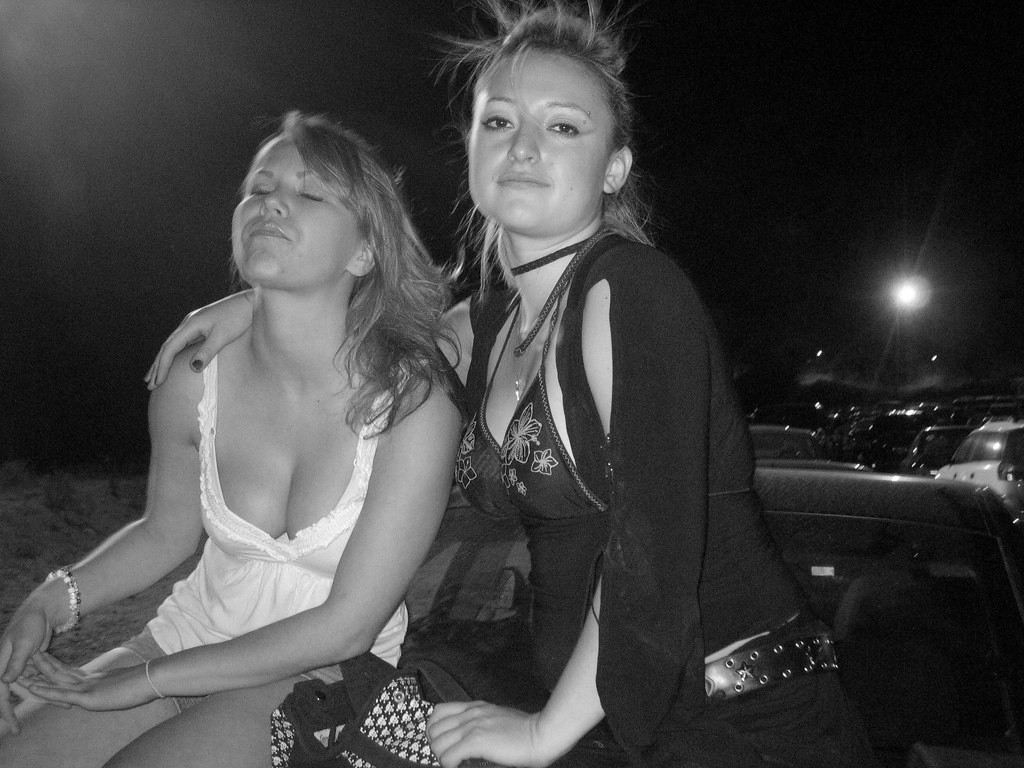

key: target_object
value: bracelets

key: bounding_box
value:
[45,565,81,634]
[145,658,167,699]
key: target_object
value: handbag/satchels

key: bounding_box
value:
[270,656,501,768]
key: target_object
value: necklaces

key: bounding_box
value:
[515,314,550,400]
[509,226,608,359]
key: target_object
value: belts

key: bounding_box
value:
[704,632,840,700]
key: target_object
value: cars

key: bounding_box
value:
[753,467,1019,756]
[751,389,1024,515]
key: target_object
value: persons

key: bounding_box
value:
[0,111,464,768]
[143,0,872,768]
